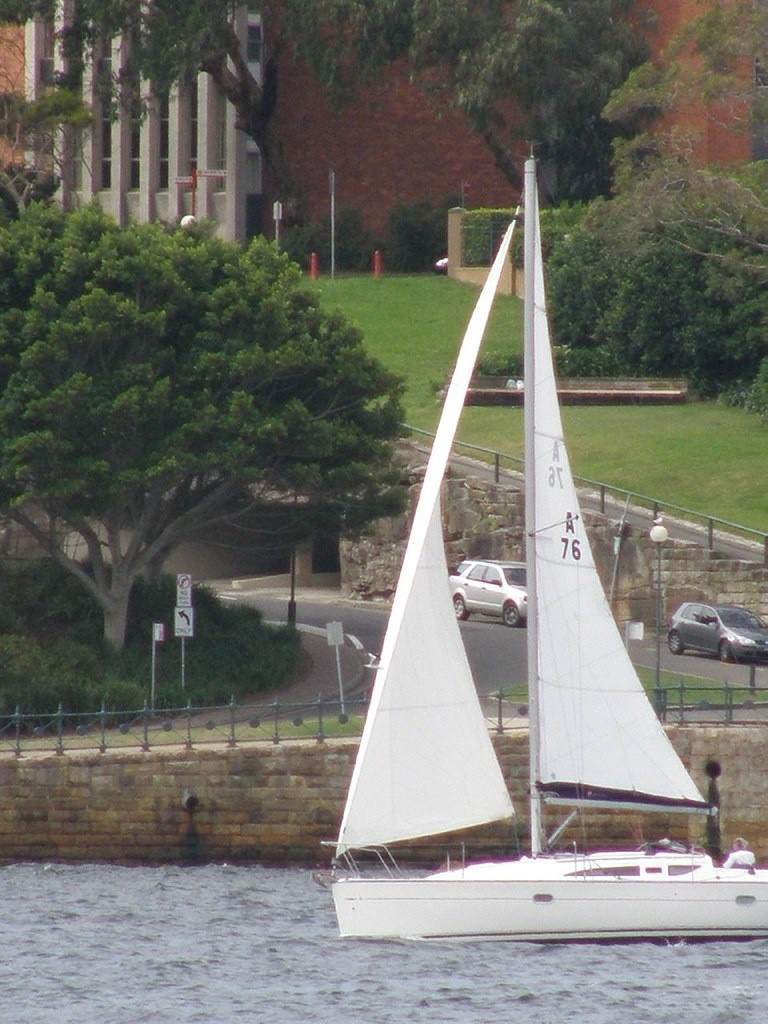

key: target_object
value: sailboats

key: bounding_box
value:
[318,131,768,947]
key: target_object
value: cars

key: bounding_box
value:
[666,600,768,665]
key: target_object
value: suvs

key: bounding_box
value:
[448,558,529,628]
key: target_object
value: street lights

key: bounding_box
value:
[650,525,670,691]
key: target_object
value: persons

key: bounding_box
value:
[723,837,755,869]
